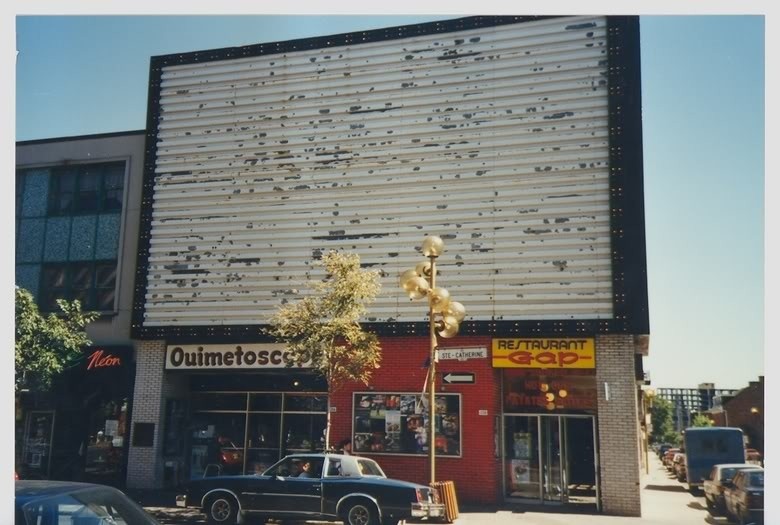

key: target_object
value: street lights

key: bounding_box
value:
[400,234,466,488]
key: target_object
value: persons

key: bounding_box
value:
[298,462,313,478]
[337,438,351,455]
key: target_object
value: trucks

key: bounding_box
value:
[681,428,746,493]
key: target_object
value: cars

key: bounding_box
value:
[722,469,764,525]
[174,453,446,525]
[652,442,686,482]
[700,464,763,515]
[744,448,762,466]
[15,479,162,525]
